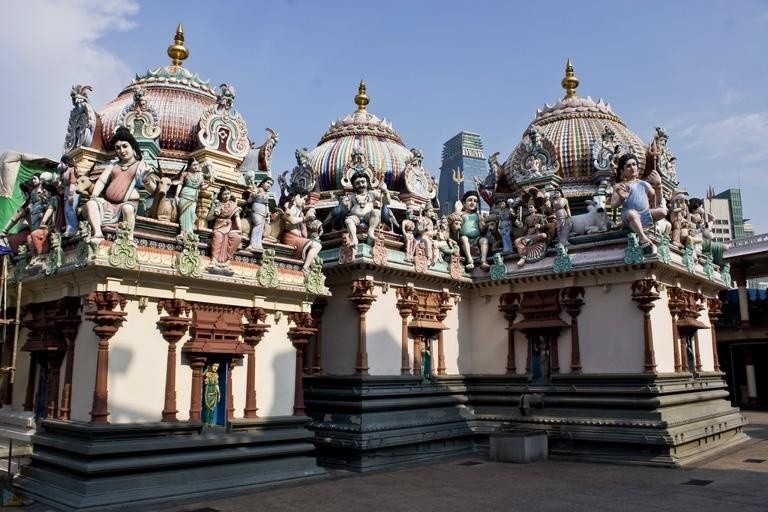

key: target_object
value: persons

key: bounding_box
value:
[3,126,727,279]
[534,334,551,379]
[420,336,432,376]
[203,356,224,429]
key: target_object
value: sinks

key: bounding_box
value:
[488,428,549,464]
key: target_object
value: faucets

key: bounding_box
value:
[517,393,544,416]
